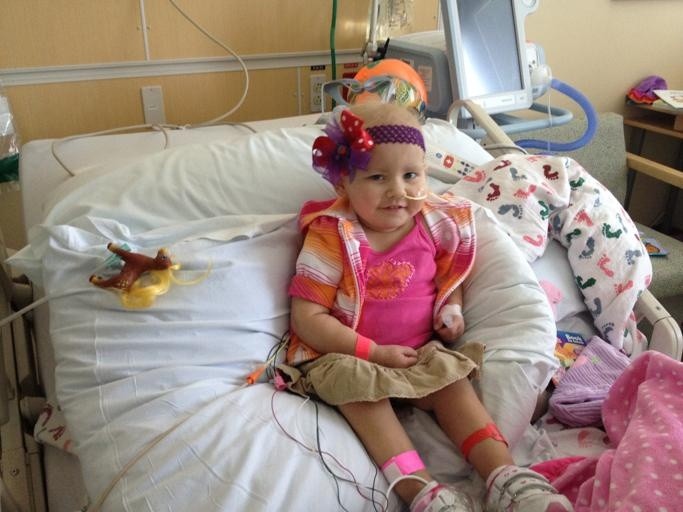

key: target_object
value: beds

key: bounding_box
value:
[19,102,682,512]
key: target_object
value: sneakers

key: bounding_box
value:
[408,481,473,512]
[482,463,574,512]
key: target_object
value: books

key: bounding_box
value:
[652,87,682,110]
[550,325,587,389]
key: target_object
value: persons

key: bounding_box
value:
[277,97,575,511]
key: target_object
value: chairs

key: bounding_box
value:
[482,112,683,330]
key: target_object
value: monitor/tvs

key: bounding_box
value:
[441,0,534,118]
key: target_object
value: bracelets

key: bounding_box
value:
[353,330,370,361]
